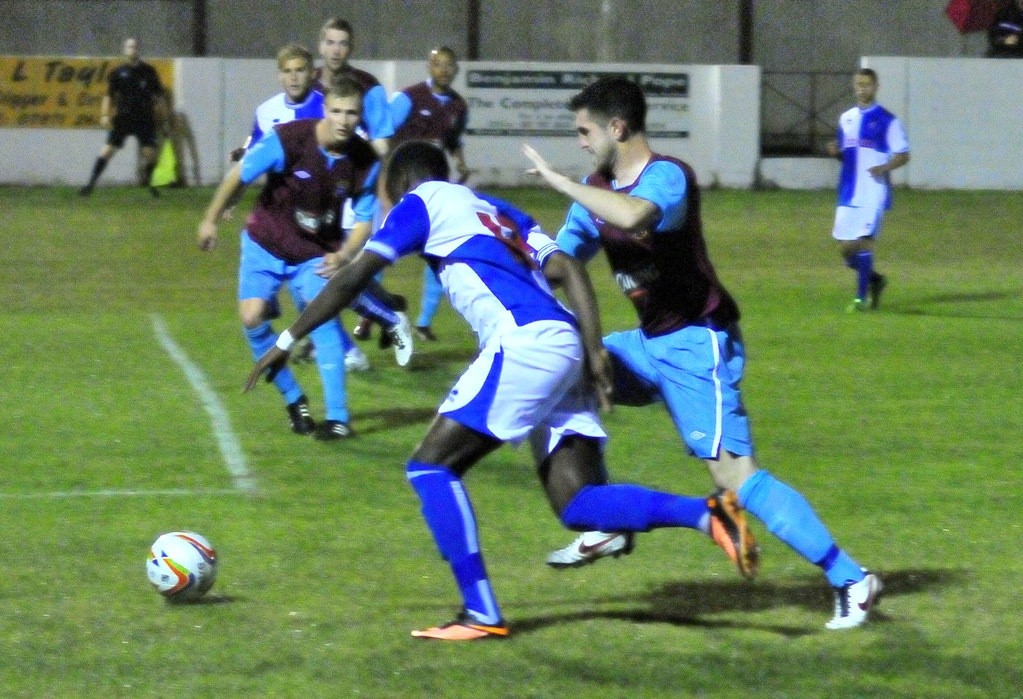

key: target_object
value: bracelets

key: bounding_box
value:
[275,329,299,351]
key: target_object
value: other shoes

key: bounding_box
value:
[846,301,868,316]
[76,187,92,198]
[413,325,437,343]
[354,315,373,343]
[138,182,160,203]
[378,293,408,349]
[868,275,885,309]
[343,349,374,373]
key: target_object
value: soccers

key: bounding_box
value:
[145,529,219,603]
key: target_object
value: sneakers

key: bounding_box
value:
[545,528,634,567]
[409,607,512,642]
[385,310,415,368]
[313,420,355,441]
[824,566,884,629]
[283,394,317,436]
[705,487,759,581]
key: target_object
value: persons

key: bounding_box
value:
[80,37,170,197]
[240,140,760,645]
[826,68,912,314]
[197,15,471,444]
[521,75,887,634]
